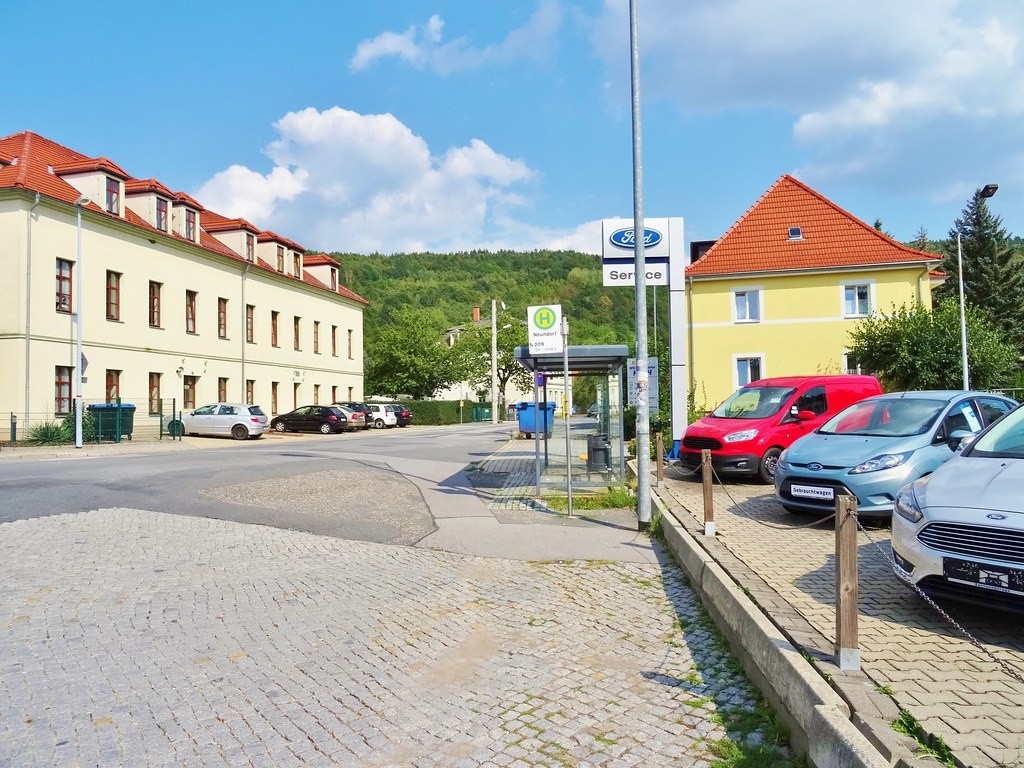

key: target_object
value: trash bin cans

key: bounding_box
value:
[516,401,556,439]
[86,403,136,441]
[586,433,609,471]
[572,404,577,415]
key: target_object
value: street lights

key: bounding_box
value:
[953,185,998,390]
[241,265,250,404]
[73,192,92,448]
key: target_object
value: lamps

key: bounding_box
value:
[789,227,802,240]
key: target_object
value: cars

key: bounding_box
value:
[366,404,397,429]
[271,405,348,434]
[890,399,1024,616]
[390,404,413,427]
[331,402,374,430]
[774,391,1020,517]
[162,402,270,439]
[330,405,366,432]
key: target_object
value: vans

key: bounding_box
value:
[678,376,883,484]
